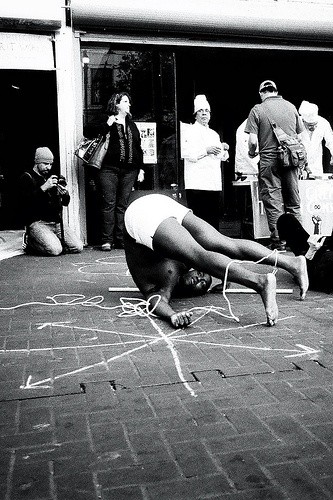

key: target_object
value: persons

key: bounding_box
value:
[181,94,230,234]
[20,146,84,257]
[234,115,261,183]
[243,80,307,254]
[298,100,333,179]
[92,92,145,251]
[122,194,310,327]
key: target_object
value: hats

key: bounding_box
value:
[35,147,53,164]
[259,80,277,92]
[299,100,318,124]
[194,94,210,114]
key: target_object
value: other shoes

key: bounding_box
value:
[265,243,287,253]
[102,243,111,251]
[21,226,30,254]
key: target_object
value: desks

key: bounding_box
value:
[233,179,333,239]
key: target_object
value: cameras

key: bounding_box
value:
[53,175,67,187]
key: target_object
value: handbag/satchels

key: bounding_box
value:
[278,135,307,167]
[73,115,112,168]
